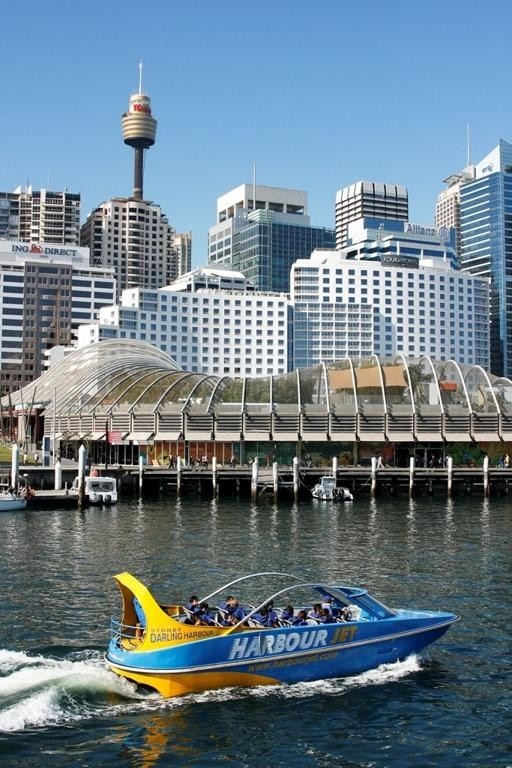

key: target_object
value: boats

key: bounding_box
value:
[70,476,118,504]
[104,688,364,768]
[313,498,353,514]
[104,571,460,699]
[310,476,354,500]
[0,484,28,511]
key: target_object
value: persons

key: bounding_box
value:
[189,594,332,626]
[24,452,28,465]
[35,451,38,466]
[498,453,511,467]
[7,483,34,501]
[248,455,253,470]
[429,452,450,468]
[162,454,208,470]
[229,452,238,469]
[306,454,312,468]
[377,454,385,469]
